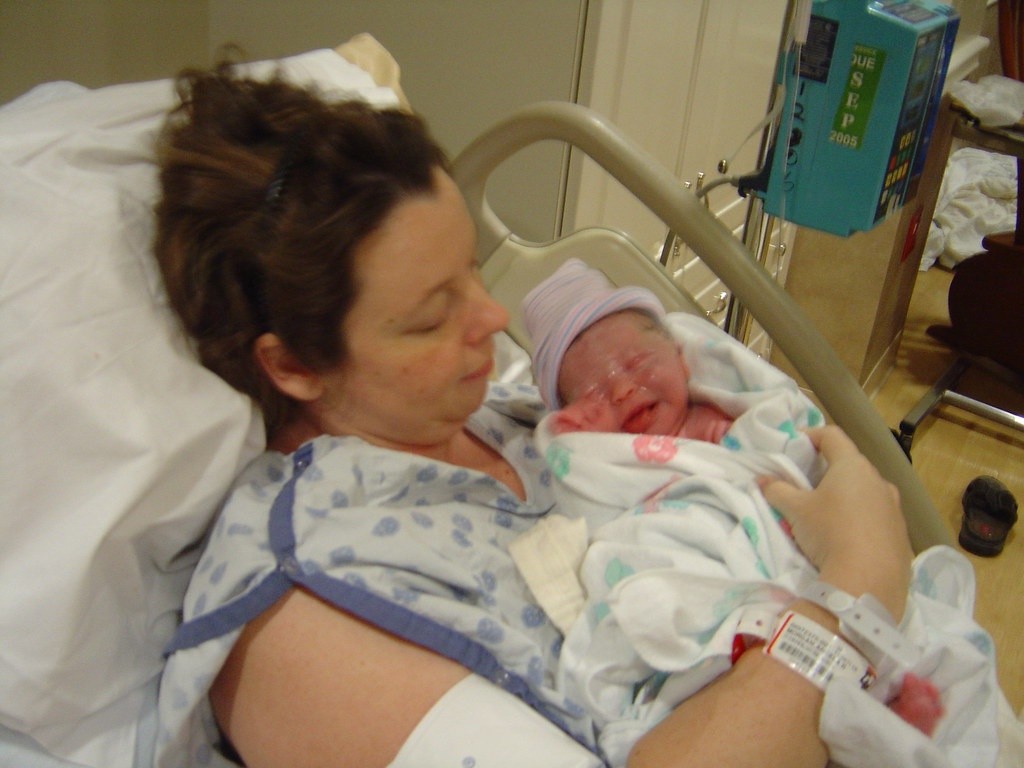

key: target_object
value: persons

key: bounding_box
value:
[145,46,915,767]
[521,257,998,768]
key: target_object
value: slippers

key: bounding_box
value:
[957,473,1018,557]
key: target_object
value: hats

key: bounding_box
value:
[524,256,666,411]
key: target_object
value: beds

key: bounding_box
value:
[0,33,1024,767]
